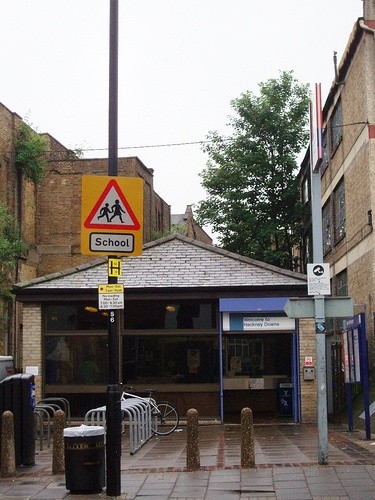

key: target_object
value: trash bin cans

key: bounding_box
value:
[66,426,108,496]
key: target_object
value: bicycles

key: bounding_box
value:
[119,382,179,435]
